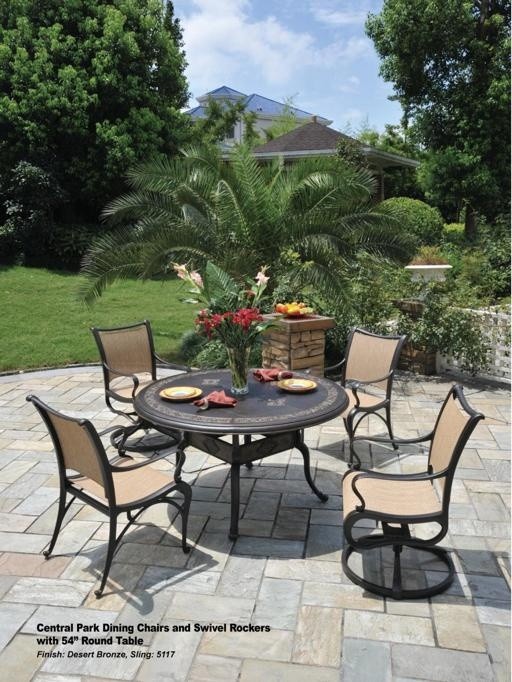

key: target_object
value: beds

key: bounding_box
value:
[132,367,350,539]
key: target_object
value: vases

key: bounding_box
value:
[225,345,251,395]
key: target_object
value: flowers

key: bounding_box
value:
[172,260,289,388]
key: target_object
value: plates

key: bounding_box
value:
[159,387,202,401]
[278,379,317,392]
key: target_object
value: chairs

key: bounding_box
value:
[323,326,408,469]
[26,395,193,597]
[89,320,181,450]
[341,382,486,599]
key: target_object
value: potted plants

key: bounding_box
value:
[395,300,488,375]
[404,244,452,281]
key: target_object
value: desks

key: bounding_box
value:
[258,312,335,379]
